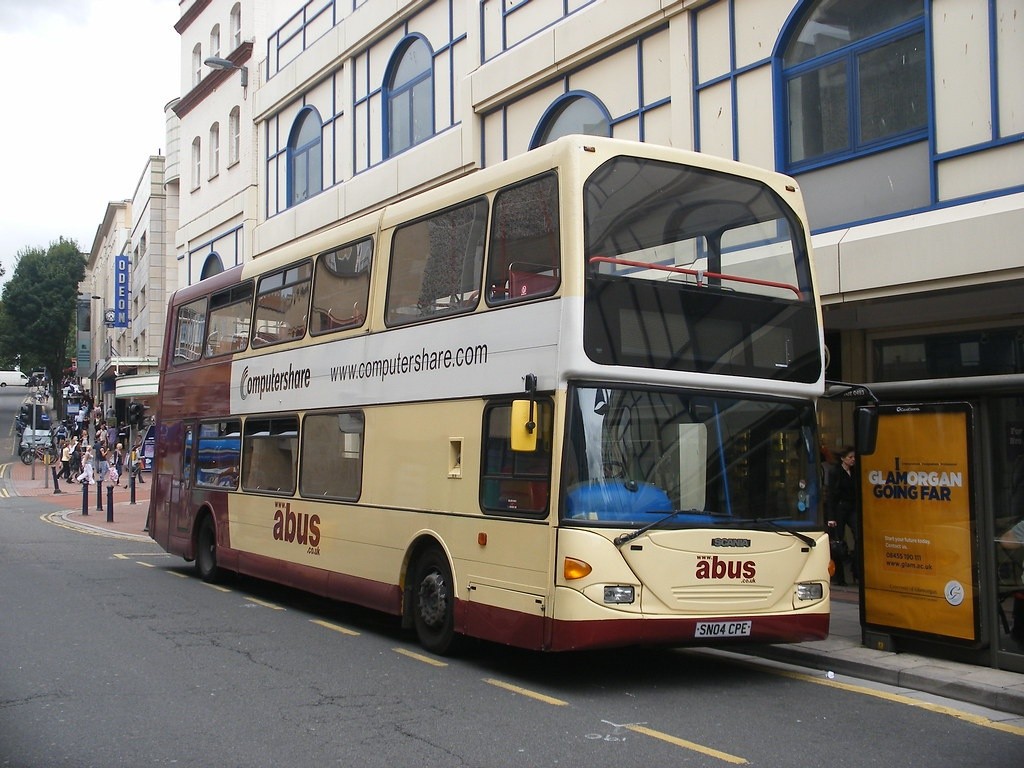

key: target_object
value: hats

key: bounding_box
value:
[132,444,137,450]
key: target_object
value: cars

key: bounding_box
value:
[15,411,51,434]
[17,425,54,455]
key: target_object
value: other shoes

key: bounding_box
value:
[66,478,75,483]
[141,481,146,483]
[118,483,121,486]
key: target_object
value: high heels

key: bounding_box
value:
[837,576,846,586]
[852,570,864,581]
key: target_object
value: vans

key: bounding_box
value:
[0,370,29,388]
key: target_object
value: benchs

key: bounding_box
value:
[206,262,560,357]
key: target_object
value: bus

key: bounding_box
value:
[149,132,881,658]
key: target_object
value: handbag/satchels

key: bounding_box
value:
[110,466,118,481]
[832,540,847,560]
[140,461,145,469]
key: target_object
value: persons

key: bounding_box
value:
[27,373,146,488]
[826,445,861,587]
[1000,520,1024,653]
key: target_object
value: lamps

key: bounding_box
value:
[203,58,249,101]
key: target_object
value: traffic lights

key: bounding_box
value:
[138,404,150,430]
[129,402,141,426]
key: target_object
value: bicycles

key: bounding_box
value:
[20,438,59,466]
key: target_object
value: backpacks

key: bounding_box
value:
[59,427,65,433]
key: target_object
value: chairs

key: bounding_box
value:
[501,468,547,514]
